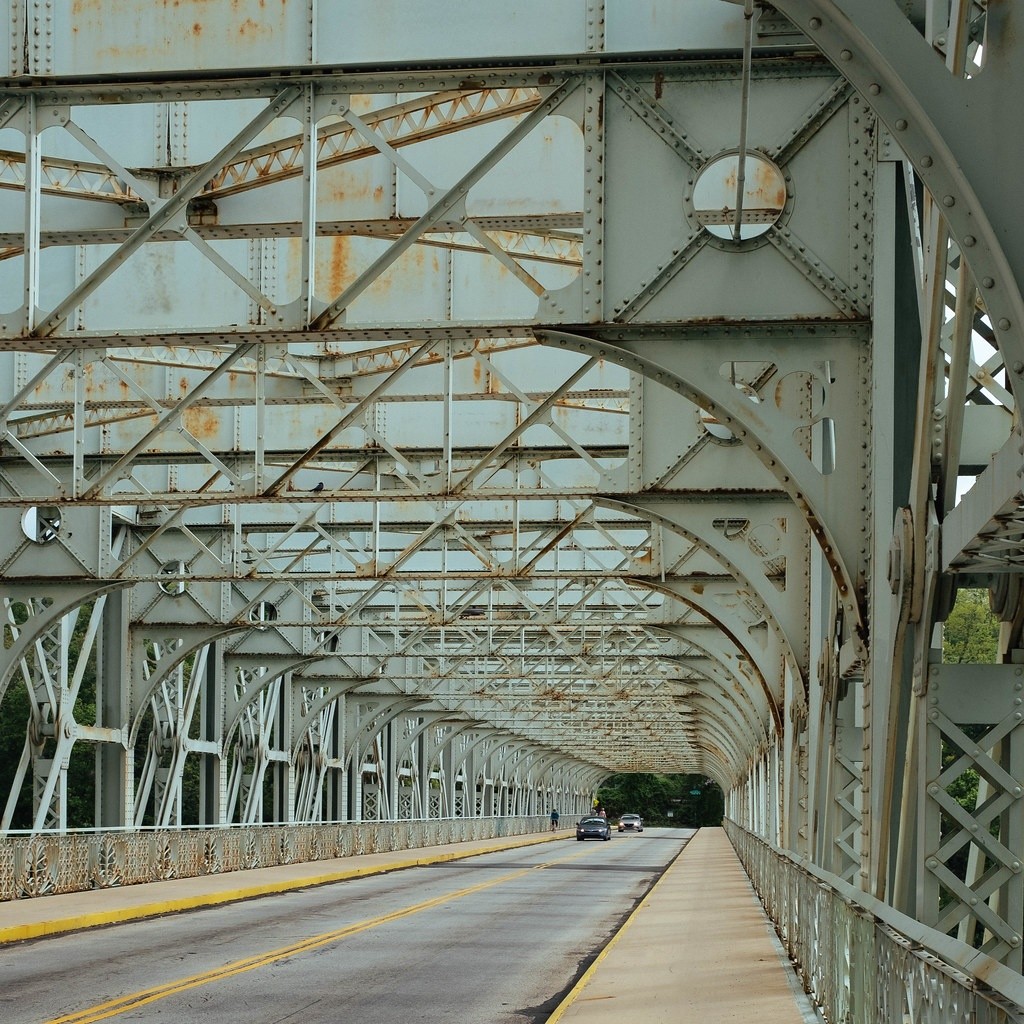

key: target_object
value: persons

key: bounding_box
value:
[551,809,559,831]
[591,807,606,818]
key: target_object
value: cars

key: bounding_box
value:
[617,814,644,833]
[576,816,611,841]
